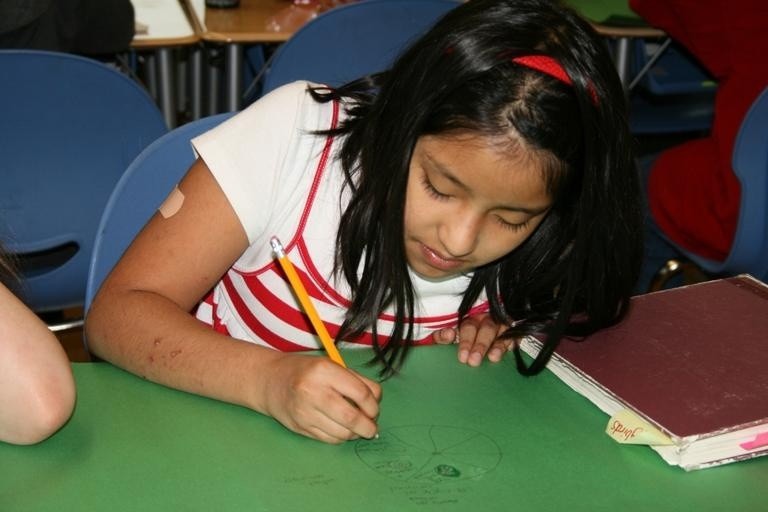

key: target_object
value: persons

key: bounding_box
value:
[78,0,650,448]
[1,241,79,448]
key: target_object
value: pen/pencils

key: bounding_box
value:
[270,235,380,439]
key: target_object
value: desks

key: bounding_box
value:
[0,335,768,512]
[592,16,677,93]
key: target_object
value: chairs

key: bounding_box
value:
[1,3,459,323]
[642,86,768,293]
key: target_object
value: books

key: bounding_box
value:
[510,272,768,475]
